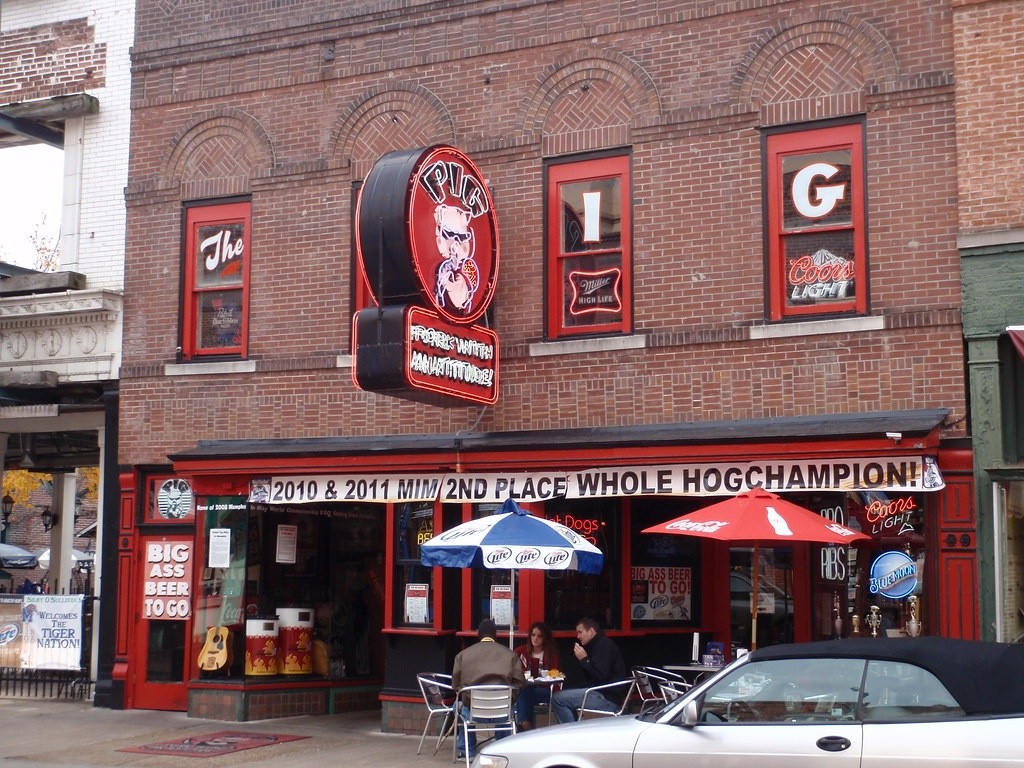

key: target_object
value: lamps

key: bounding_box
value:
[0,490,14,529]
[74,496,83,523]
[41,505,58,532]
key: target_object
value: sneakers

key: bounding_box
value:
[456,751,475,762]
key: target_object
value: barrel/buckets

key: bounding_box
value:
[245,619,280,676]
[276,607,314,675]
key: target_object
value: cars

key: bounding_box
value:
[730,570,794,645]
[468,636,1024,768]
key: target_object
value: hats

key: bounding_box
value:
[479,618,496,633]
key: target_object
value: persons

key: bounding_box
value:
[451,618,525,763]
[550,615,630,724]
[512,621,565,734]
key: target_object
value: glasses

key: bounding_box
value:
[531,633,542,638]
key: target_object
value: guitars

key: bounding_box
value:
[195,583,233,670]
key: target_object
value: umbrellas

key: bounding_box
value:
[0,543,38,570]
[640,486,871,654]
[419,498,604,652]
[37,548,93,570]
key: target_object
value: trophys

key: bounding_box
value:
[831,541,922,638]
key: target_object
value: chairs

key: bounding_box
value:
[575,677,638,721]
[416,672,452,755]
[631,663,689,713]
[657,681,693,704]
[453,685,520,768]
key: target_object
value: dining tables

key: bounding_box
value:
[663,665,727,687]
[518,669,566,726]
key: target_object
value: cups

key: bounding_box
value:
[531,657,539,675]
